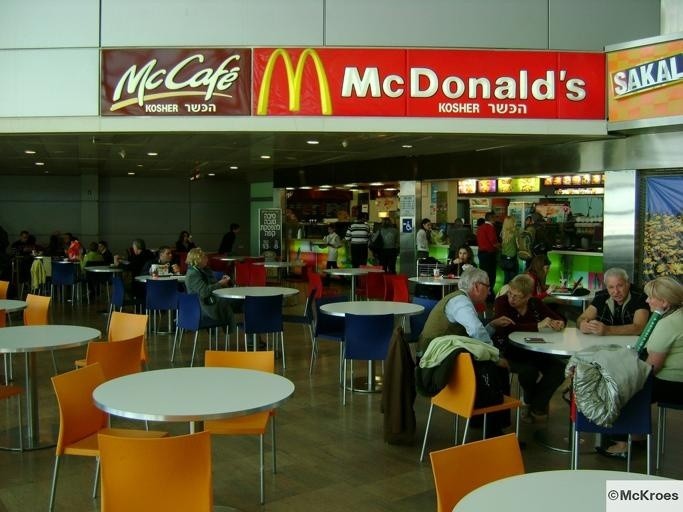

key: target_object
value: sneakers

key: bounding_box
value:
[518,398,536,424]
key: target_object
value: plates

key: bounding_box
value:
[314,242,328,244]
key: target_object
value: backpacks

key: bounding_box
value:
[518,225,542,260]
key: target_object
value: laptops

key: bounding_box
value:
[550,276,584,296]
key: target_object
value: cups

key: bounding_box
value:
[433,269,440,279]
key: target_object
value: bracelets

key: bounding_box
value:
[539,322,543,332]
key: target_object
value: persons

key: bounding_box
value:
[372,218,400,274]
[142,245,181,274]
[449,218,470,259]
[176,230,196,252]
[521,254,556,298]
[99,240,114,265]
[526,216,535,228]
[49,232,78,257]
[418,267,527,451]
[500,216,521,285]
[11,231,42,257]
[594,277,683,460]
[345,213,370,268]
[126,238,156,300]
[320,225,346,280]
[417,218,432,258]
[525,213,553,286]
[185,248,266,347]
[548,218,553,224]
[448,245,477,277]
[80,242,103,269]
[577,268,651,336]
[494,274,568,424]
[22,235,37,255]
[219,222,241,255]
[477,212,500,305]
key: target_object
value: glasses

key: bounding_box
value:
[479,282,490,289]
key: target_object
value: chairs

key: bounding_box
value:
[305,296,350,360]
[234,295,287,369]
[306,269,337,300]
[567,364,652,476]
[203,350,276,505]
[341,313,395,406]
[0,308,13,385]
[655,392,683,470]
[383,274,410,302]
[48,361,170,511]
[355,265,383,301]
[248,264,281,287]
[145,278,178,335]
[74,335,149,431]
[282,288,319,358]
[365,271,385,302]
[0,386,25,453]
[50,261,80,306]
[106,277,140,332]
[430,433,526,512]
[0,280,11,327]
[74,311,149,371]
[234,262,251,287]
[170,292,229,367]
[98,430,212,512]
[409,297,438,337]
[23,293,55,361]
[418,351,522,463]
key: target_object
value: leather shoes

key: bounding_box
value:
[631,439,648,446]
[596,444,628,461]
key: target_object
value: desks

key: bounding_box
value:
[451,469,683,512]
[92,366,296,436]
[212,286,299,359]
[211,255,265,262]
[508,327,641,454]
[322,268,385,302]
[429,245,603,294]
[83,265,123,314]
[319,300,425,393]
[553,288,603,312]
[287,238,400,274]
[253,261,305,268]
[0,325,102,452]
[135,275,186,335]
[0,299,28,313]
[408,275,460,299]
